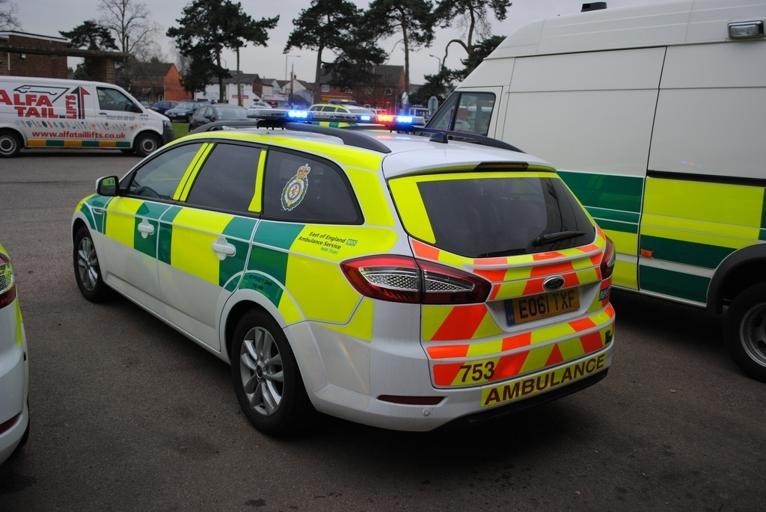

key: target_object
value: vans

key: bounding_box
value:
[0,76,174,156]
[310,97,374,127]
[420,0,766,384]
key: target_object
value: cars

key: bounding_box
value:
[152,98,250,130]
[71,106,616,435]
[0,244,32,463]
